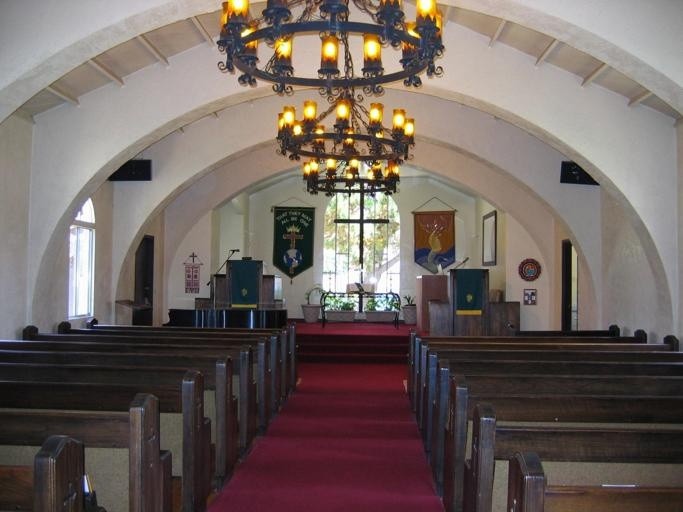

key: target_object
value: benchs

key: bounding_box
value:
[405,325,683,512]
[0,319,301,512]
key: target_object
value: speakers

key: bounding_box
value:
[107,159,152,181]
[559,160,600,186]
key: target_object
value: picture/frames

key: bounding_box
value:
[523,289,536,305]
[482,210,497,266]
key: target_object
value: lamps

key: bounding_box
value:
[215,0,443,98]
[303,143,399,198]
[275,87,416,161]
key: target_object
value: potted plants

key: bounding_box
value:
[364,295,397,323]
[300,284,328,323]
[323,294,357,322]
[401,294,417,325]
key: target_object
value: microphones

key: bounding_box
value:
[454,257,469,269]
[231,249,240,252]
